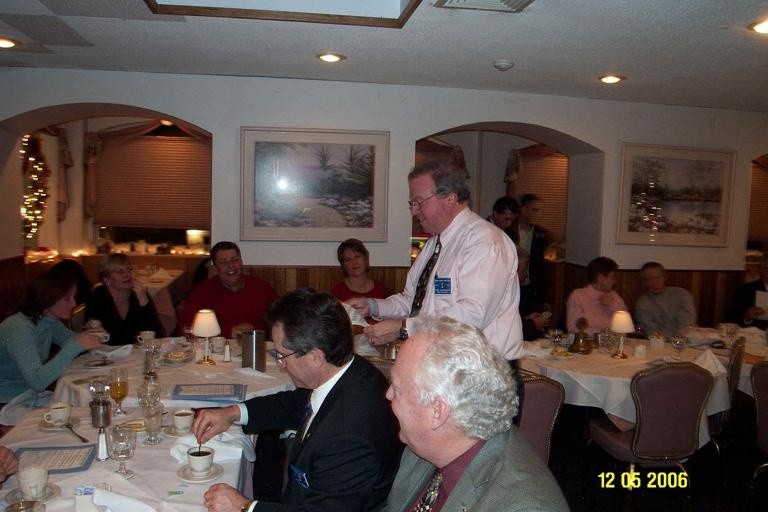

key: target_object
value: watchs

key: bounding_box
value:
[239,498,253,511]
[400,318,406,339]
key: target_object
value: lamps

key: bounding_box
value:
[607,310,635,359]
[191,309,221,365]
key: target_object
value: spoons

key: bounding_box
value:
[63,423,89,442]
[198,443,202,456]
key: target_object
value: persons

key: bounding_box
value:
[513,195,548,312]
[349,158,525,429]
[184,241,280,339]
[331,239,391,379]
[1,271,102,410]
[0,445,18,483]
[193,287,405,512]
[513,244,551,340]
[726,254,768,327]
[85,253,164,346]
[484,197,519,232]
[635,262,697,342]
[383,317,570,511]
[566,257,628,335]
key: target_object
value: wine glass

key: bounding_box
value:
[109,427,136,479]
[138,331,155,343]
[671,337,686,361]
[110,368,128,414]
[727,323,738,347]
[144,406,162,445]
[552,329,562,348]
[89,331,110,343]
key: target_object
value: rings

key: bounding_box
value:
[95,341,98,344]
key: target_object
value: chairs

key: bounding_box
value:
[518,367,565,463]
[587,361,713,498]
[365,358,396,385]
[711,337,745,455]
[750,361,767,488]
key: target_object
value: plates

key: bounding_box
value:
[552,351,577,360]
[164,351,192,363]
[38,416,81,431]
[6,483,61,504]
[177,463,223,483]
[84,357,114,369]
[164,424,194,438]
[119,418,145,431]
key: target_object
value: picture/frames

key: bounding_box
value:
[239,126,390,242]
[615,142,737,247]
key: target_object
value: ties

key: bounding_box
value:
[412,470,444,512]
[281,390,312,498]
[410,235,443,318]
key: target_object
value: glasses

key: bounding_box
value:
[408,190,447,206]
[276,350,303,365]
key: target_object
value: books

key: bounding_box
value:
[754,290,768,320]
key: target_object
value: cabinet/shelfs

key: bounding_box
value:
[82,248,210,298]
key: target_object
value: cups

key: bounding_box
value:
[44,402,72,426]
[173,409,195,434]
[242,330,266,372]
[187,446,214,477]
[210,337,226,353]
[17,467,49,500]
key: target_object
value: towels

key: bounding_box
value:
[526,341,551,357]
[170,428,256,463]
[92,489,157,512]
[694,348,726,375]
